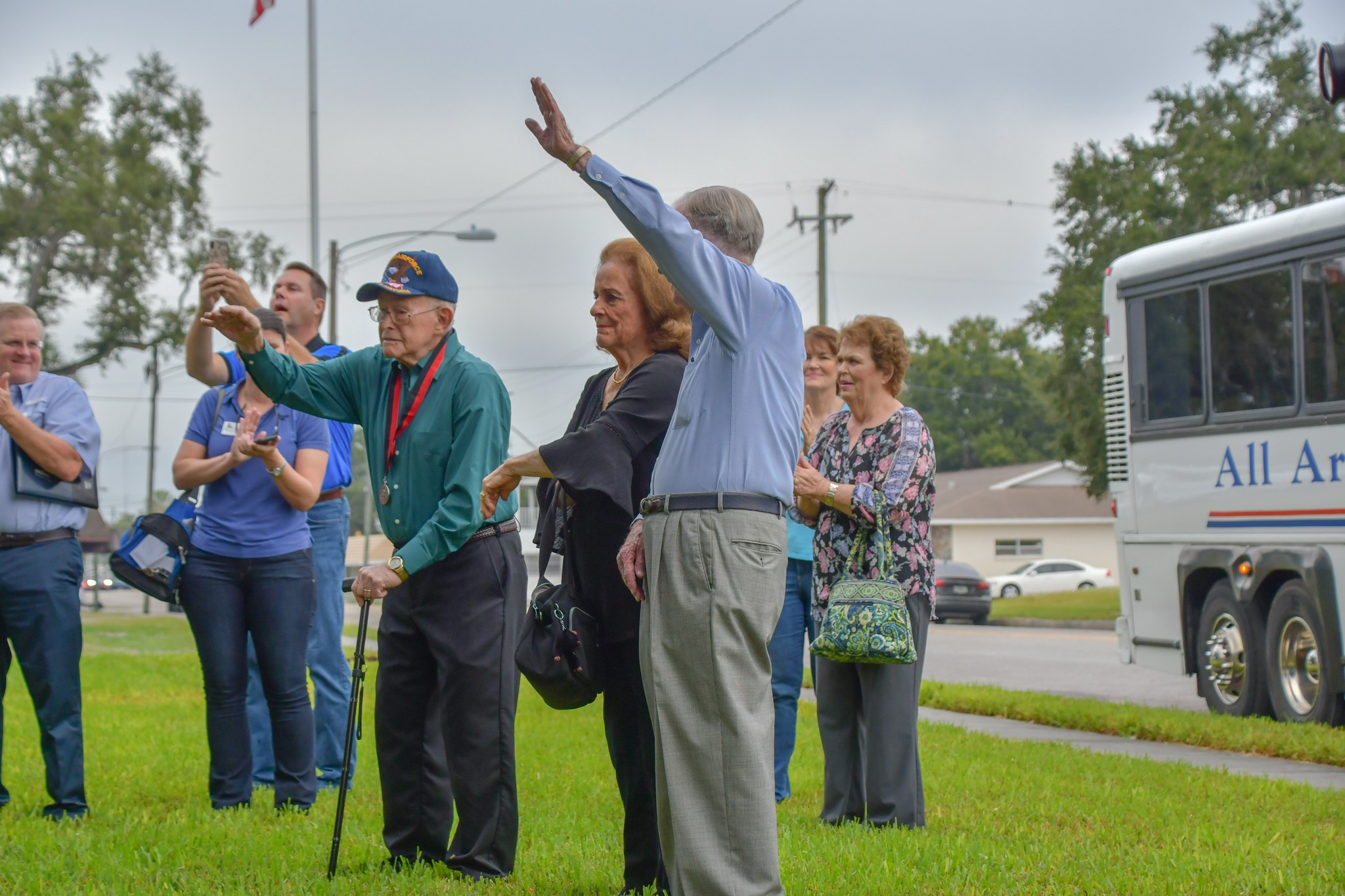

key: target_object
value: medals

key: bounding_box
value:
[379,481,390,505]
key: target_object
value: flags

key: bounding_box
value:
[248,0,275,25]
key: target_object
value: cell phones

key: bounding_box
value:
[255,434,278,445]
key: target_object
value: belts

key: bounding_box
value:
[638,491,786,518]
[0,527,76,552]
[465,517,517,544]
[315,485,345,506]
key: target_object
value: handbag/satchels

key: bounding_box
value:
[513,479,608,710]
[111,512,192,607]
[805,489,927,666]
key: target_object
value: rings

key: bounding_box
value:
[480,491,486,498]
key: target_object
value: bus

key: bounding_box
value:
[1096,192,1345,733]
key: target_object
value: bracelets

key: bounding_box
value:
[364,589,371,592]
[826,482,839,506]
[566,146,592,171]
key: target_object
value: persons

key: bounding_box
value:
[524,72,784,896]
[186,260,361,794]
[0,300,100,825]
[172,308,330,819]
[767,325,851,803]
[200,250,528,884]
[791,312,939,829]
[476,240,690,896]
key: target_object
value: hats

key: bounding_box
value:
[355,249,458,304]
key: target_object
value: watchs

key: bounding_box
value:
[388,556,408,581]
[266,458,286,477]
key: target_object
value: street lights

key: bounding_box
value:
[329,225,498,345]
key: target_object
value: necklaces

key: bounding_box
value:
[613,351,656,383]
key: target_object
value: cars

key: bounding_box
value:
[935,560,991,625]
[984,558,1114,599]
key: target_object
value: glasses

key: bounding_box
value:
[368,305,440,326]
